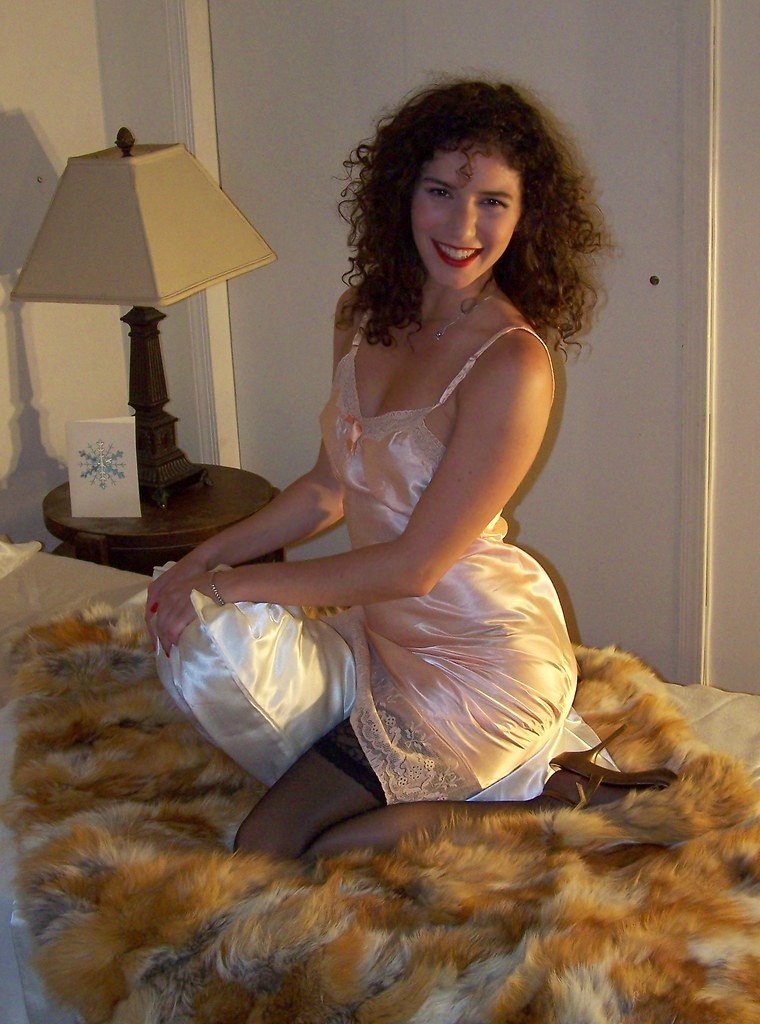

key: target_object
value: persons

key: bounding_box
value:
[145,81,679,864]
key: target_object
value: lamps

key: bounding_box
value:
[7,126,280,513]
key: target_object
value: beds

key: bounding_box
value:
[0,529,760,1024]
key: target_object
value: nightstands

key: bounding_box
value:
[41,459,286,578]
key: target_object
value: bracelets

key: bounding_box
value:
[209,567,225,605]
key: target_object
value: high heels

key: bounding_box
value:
[549,723,684,807]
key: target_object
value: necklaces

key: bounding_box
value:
[419,285,502,343]
